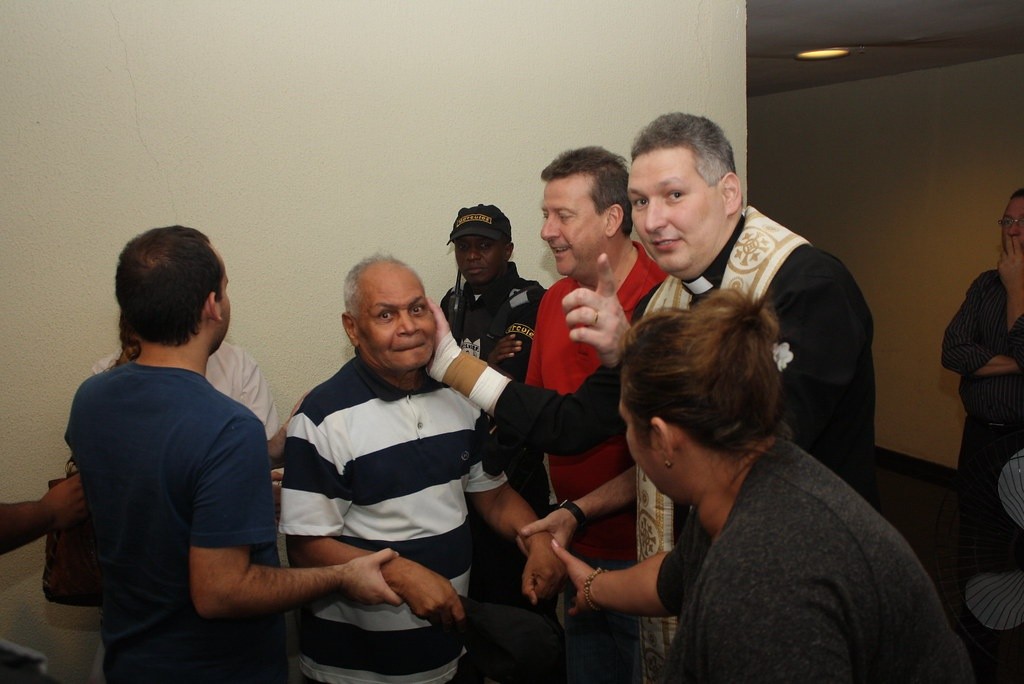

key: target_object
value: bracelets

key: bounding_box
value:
[584,567,608,610]
[555,499,587,532]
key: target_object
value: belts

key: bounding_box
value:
[970,416,1024,433]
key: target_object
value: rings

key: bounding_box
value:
[591,312,598,325]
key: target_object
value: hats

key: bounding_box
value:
[446,205,512,247]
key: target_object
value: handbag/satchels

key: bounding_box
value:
[43,452,107,605]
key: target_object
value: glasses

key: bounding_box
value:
[997,217,1024,229]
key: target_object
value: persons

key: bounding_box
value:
[93,304,280,440]
[551,287,974,684]
[940,190,1024,684]
[0,472,87,556]
[425,112,874,684]
[278,253,569,684]
[63,224,404,684]
[440,207,550,600]
[520,146,669,684]
